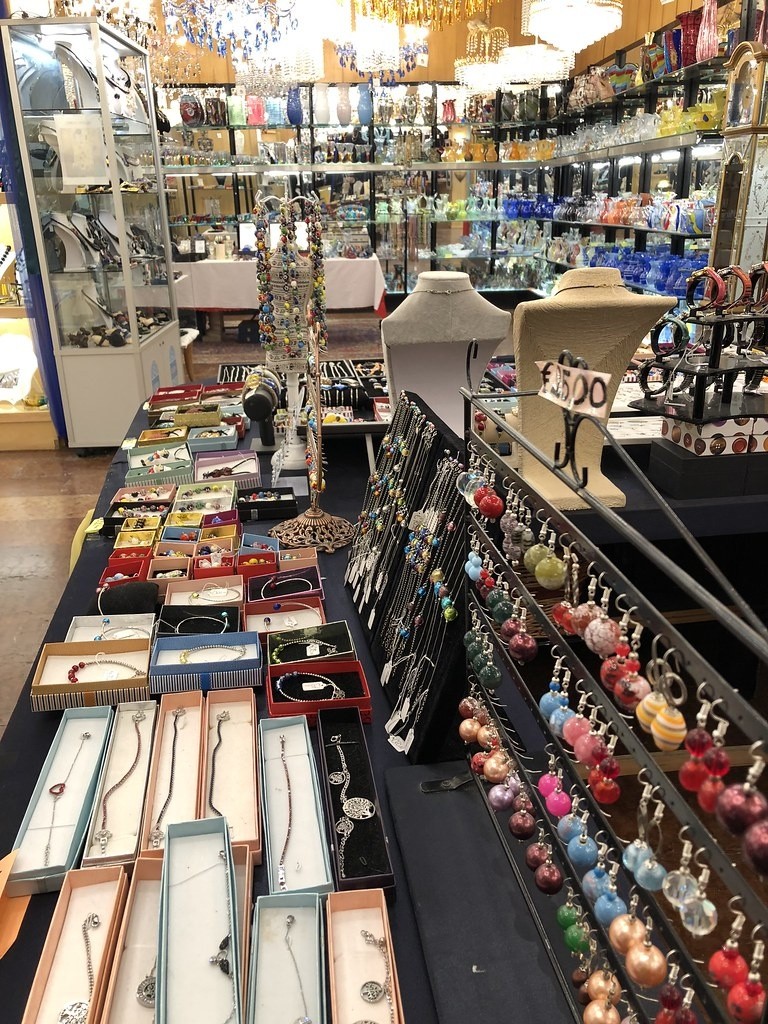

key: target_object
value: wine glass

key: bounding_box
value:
[553,112,656,158]
[161,142,256,166]
[336,143,374,166]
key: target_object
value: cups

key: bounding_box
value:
[683,210,706,232]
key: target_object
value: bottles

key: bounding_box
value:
[662,0,720,73]
[374,130,552,165]
[180,82,373,126]
[497,221,709,297]
[433,194,646,228]
[378,89,539,125]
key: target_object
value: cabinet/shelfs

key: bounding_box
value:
[128,78,565,316]
[0,20,186,449]
[533,0,768,349]
[0,190,62,453]
[626,315,768,425]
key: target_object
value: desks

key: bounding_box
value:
[0,389,768,1024]
[120,253,387,344]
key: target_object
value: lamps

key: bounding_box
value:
[58,0,626,107]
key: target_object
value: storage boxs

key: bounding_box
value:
[0,383,409,1024]
[743,453,768,496]
[661,418,753,457]
[647,439,748,499]
[749,418,768,452]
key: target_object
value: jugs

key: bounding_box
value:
[171,240,195,255]
